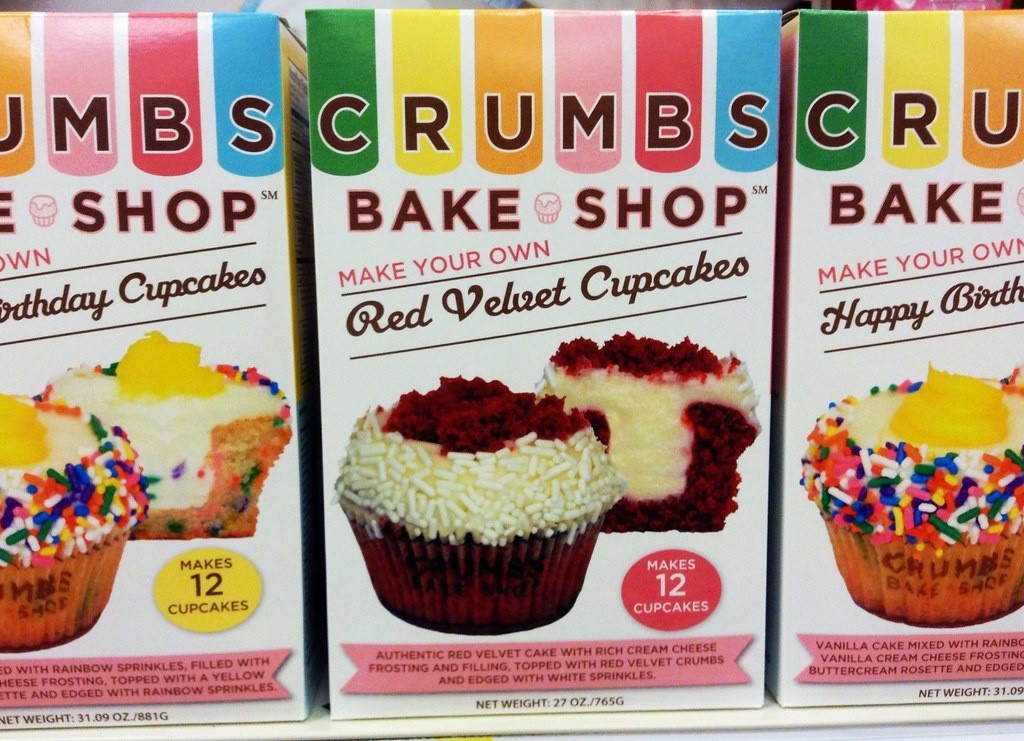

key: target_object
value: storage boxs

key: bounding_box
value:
[0,12,323,731]
[767,9,1024,708]
[303,8,782,722]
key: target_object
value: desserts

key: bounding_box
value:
[334,332,765,633]
[0,331,292,653]
[799,360,1024,629]
